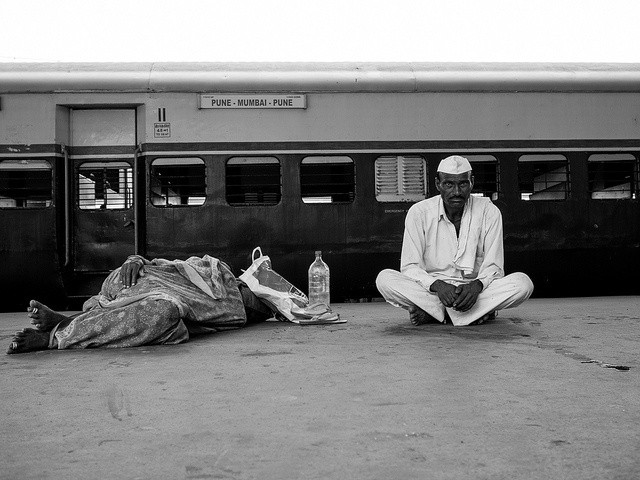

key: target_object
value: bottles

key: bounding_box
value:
[307,250,331,309]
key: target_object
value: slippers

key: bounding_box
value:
[297,312,347,325]
[291,302,341,318]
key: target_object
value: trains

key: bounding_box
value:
[0,63,640,312]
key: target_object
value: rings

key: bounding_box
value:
[12,343,17,350]
[32,308,38,314]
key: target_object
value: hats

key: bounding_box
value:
[436,154,472,175]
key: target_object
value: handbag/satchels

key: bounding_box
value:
[238,246,309,323]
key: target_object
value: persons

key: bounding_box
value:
[7,254,274,355]
[376,155,534,327]
